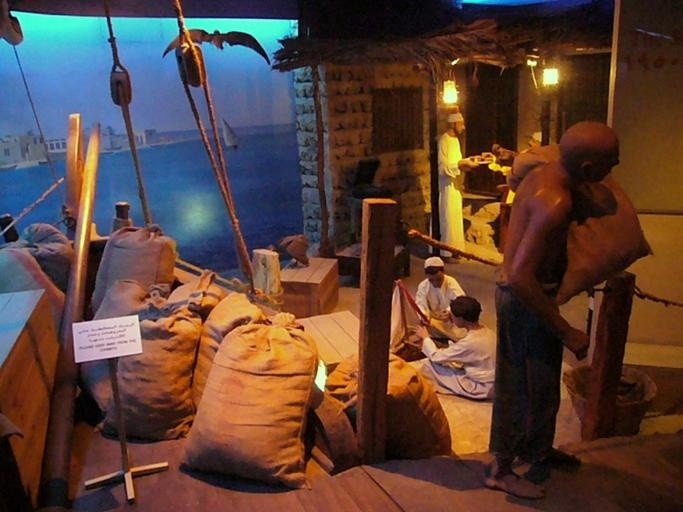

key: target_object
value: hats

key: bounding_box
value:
[448,113,463,122]
[532,132,551,144]
[424,257,444,269]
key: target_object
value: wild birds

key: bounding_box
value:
[162,28,271,66]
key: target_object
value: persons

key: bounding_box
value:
[480,130,543,210]
[414,256,467,342]
[482,119,621,501]
[436,112,484,260]
[410,292,497,402]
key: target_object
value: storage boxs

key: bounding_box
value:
[460,190,501,248]
[293,308,360,376]
[277,256,340,317]
[333,242,411,280]
[464,159,512,196]
[0,289,68,512]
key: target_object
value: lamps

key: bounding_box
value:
[442,67,459,105]
[541,54,559,86]
